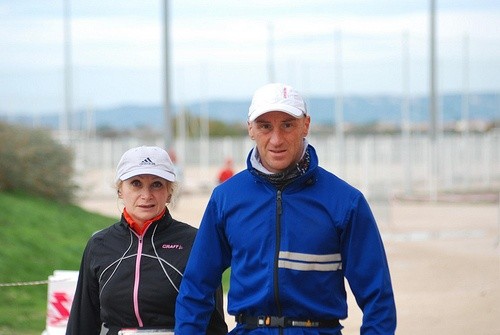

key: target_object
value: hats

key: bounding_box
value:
[246,82,307,122]
[116,145,178,184]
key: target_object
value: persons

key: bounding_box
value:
[174,83,396,335]
[66,146,229,335]
[220,161,233,184]
